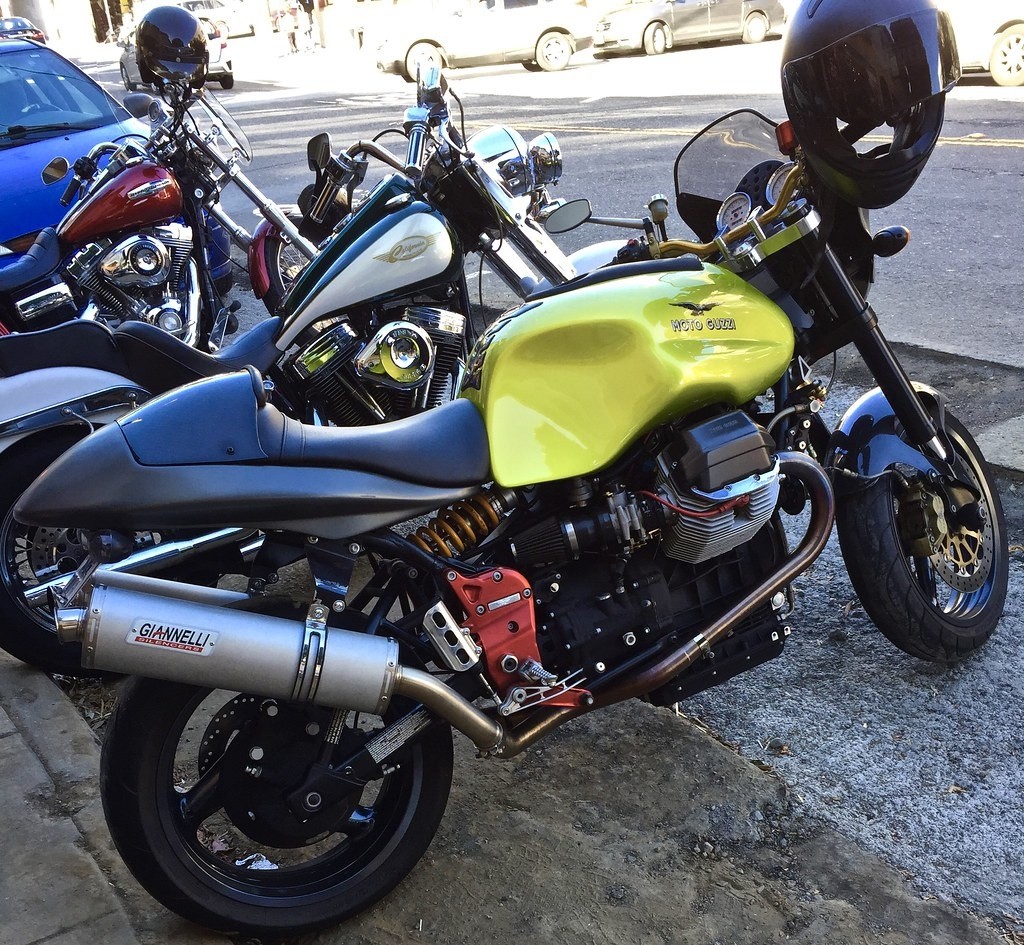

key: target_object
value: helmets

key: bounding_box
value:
[135,6,209,90]
[781,0,946,210]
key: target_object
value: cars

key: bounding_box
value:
[118,10,234,92]
[0,17,236,323]
[263,0,792,83]
[167,0,262,42]
[929,0,1024,87]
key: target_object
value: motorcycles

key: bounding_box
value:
[13,85,1011,940]
[1,71,327,371]
[1,56,661,680]
